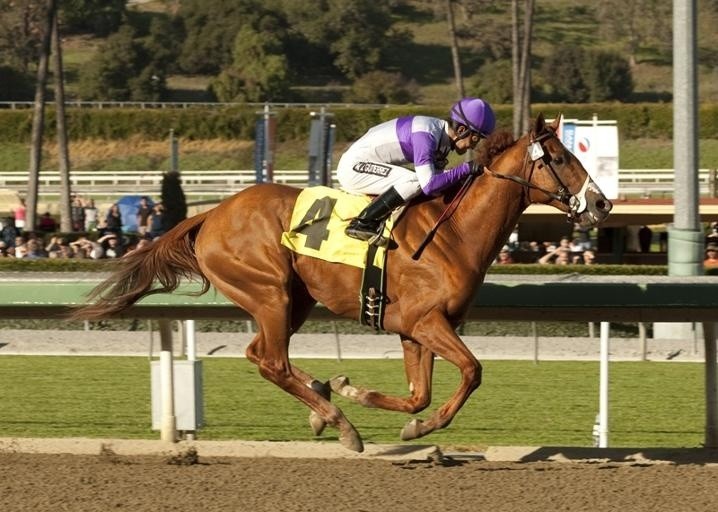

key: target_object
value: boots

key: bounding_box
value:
[345,186,407,247]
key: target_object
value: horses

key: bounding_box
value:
[62,111,613,452]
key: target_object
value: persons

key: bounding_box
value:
[338,98,495,247]
[704,222,717,265]
[639,225,652,253]
[1,197,164,259]
[493,235,598,266]
[659,232,668,251]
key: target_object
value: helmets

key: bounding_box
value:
[450,97,495,139]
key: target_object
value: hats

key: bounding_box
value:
[705,242,718,253]
